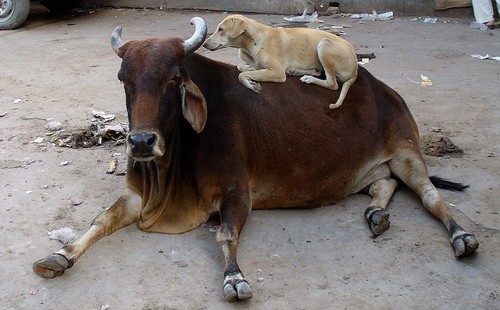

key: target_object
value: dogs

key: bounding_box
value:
[203,14,359,109]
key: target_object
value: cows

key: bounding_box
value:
[33,16,480,301]
